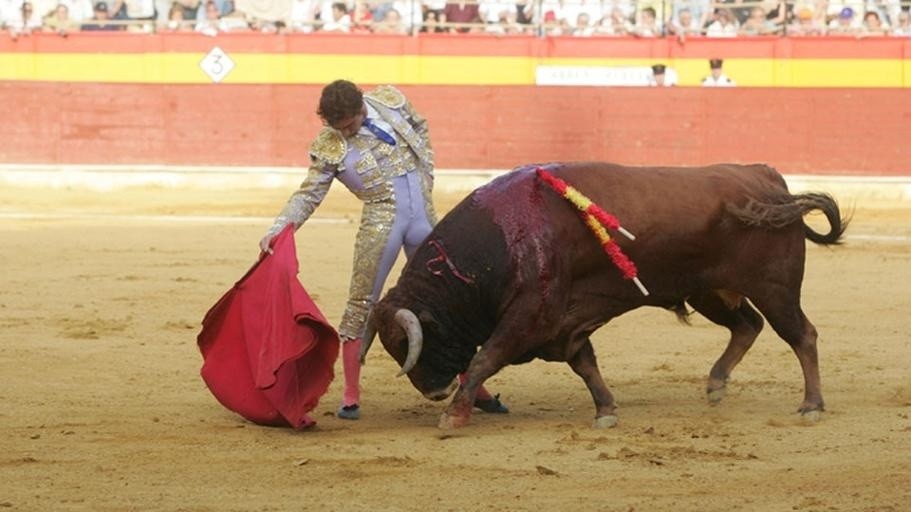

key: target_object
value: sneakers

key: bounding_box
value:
[338,403,360,420]
[474,393,511,414]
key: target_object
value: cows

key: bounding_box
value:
[360,160,857,439]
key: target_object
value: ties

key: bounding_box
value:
[362,118,398,146]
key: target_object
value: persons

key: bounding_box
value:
[257,79,509,419]
[646,65,676,86]
[700,60,735,86]
